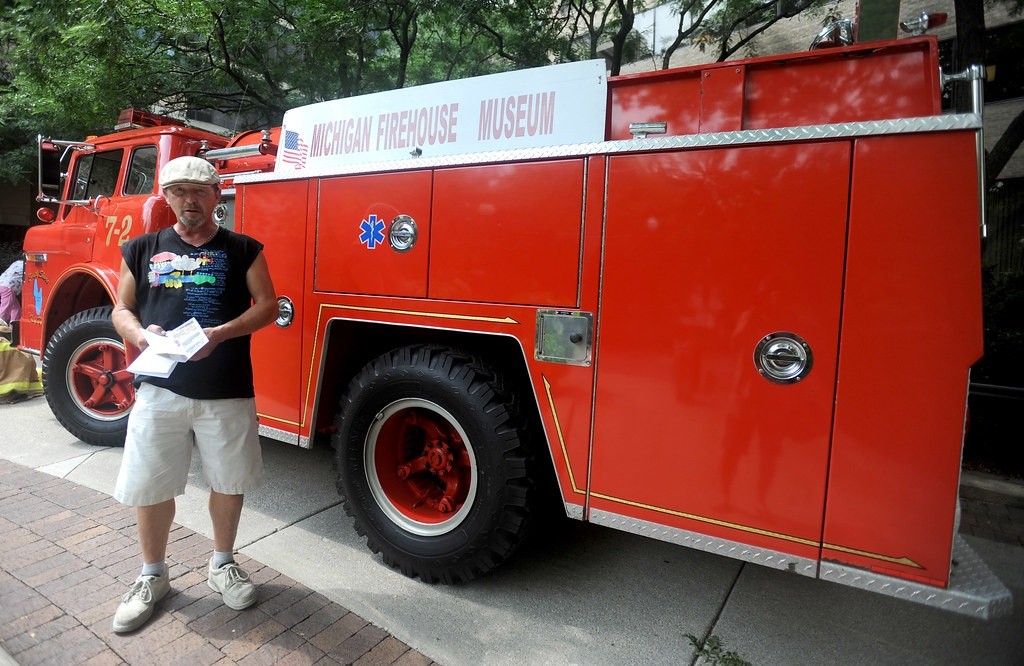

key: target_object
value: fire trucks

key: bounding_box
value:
[9,0,1024,622]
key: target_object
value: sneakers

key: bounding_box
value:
[113,563,171,633]
[207,556,258,610]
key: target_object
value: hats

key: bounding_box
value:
[158,156,220,188]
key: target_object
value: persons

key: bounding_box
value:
[0,260,23,347]
[111,156,279,635]
